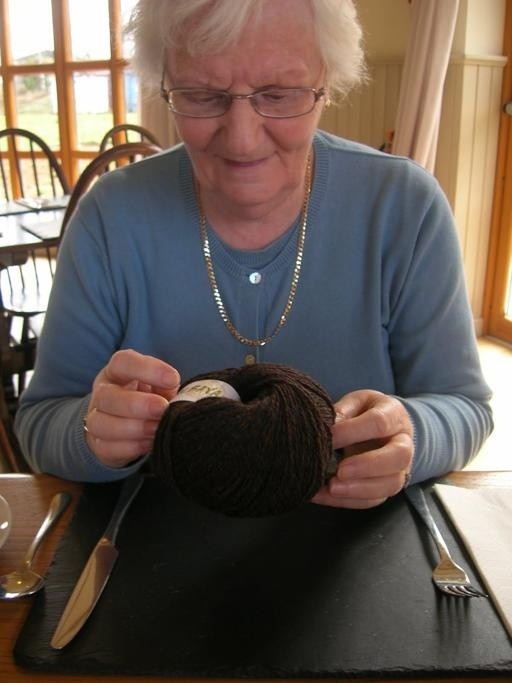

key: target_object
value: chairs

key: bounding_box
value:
[0,128,70,403]
[99,124,161,180]
[0,143,162,474]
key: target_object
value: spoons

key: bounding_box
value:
[0,490,73,602]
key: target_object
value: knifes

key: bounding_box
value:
[49,471,144,649]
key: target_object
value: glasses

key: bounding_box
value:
[159,66,329,119]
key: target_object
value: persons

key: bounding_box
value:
[12,0,493,511]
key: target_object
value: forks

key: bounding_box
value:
[401,479,493,599]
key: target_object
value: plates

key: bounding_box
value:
[0,493,13,549]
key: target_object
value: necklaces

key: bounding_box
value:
[199,148,311,347]
